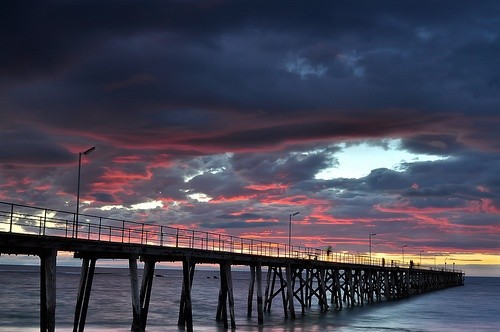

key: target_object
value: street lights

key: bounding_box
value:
[75,145,95,239]
[369,233,376,265]
[402,244,407,266]
[288,211,300,258]
[419,250,455,271]
